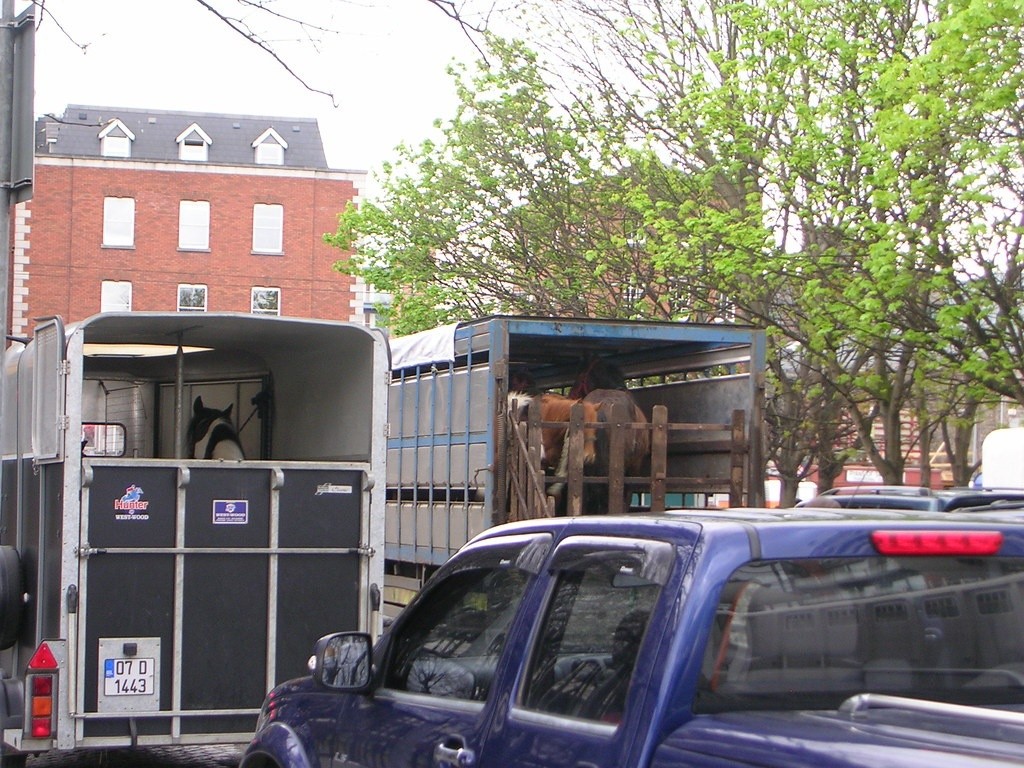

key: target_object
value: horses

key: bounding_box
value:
[570,349,650,513]
[185,393,246,459]
[509,388,602,465]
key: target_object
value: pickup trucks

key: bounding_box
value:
[236,485,1024,768]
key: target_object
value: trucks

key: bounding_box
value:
[0,310,392,754]
[382,313,767,640]
